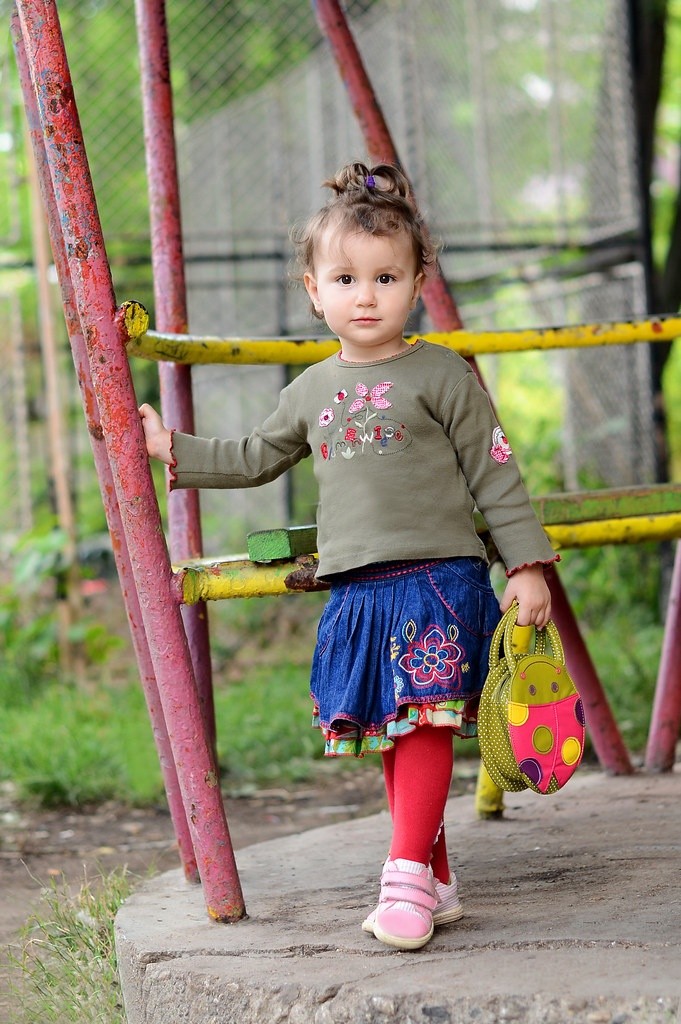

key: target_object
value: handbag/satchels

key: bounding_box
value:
[475,601,587,796]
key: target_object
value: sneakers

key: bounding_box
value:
[370,852,443,952]
[360,871,466,934]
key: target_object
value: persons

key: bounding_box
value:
[135,163,558,952]
